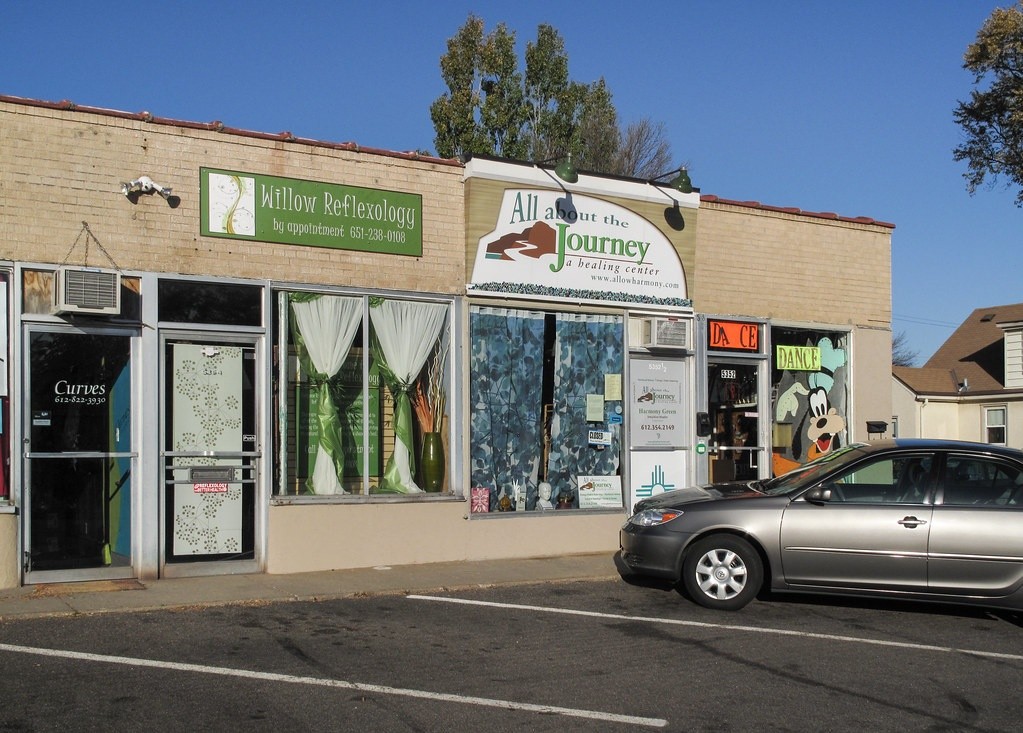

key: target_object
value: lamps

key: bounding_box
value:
[538,152,579,184]
[120,175,173,200]
[650,166,692,195]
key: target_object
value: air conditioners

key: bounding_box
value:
[52,267,122,318]
[642,317,690,349]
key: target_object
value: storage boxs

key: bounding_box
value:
[471,487,490,512]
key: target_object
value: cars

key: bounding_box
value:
[617,438,1023,612]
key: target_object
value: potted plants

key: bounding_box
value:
[408,329,452,493]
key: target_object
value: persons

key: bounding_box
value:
[732,415,750,462]
[537,482,553,510]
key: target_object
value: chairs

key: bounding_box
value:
[901,463,931,503]
